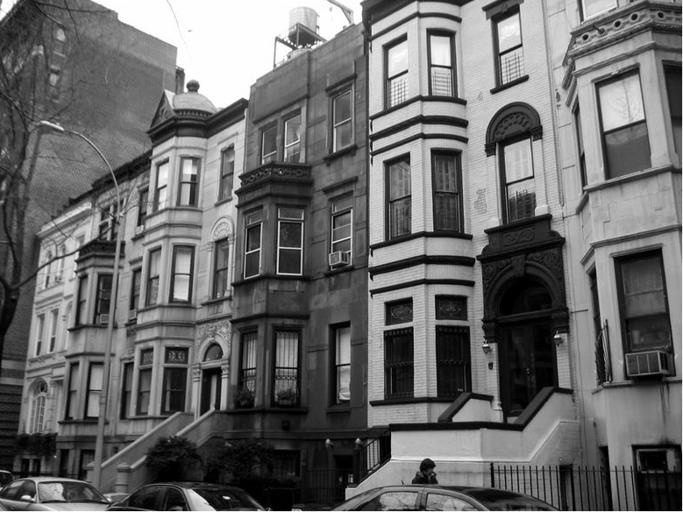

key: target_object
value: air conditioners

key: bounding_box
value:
[328,251,350,267]
[625,352,670,377]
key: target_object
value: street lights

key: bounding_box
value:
[37,119,126,490]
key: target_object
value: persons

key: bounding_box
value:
[411,458,438,485]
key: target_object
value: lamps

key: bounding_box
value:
[482,326,562,353]
